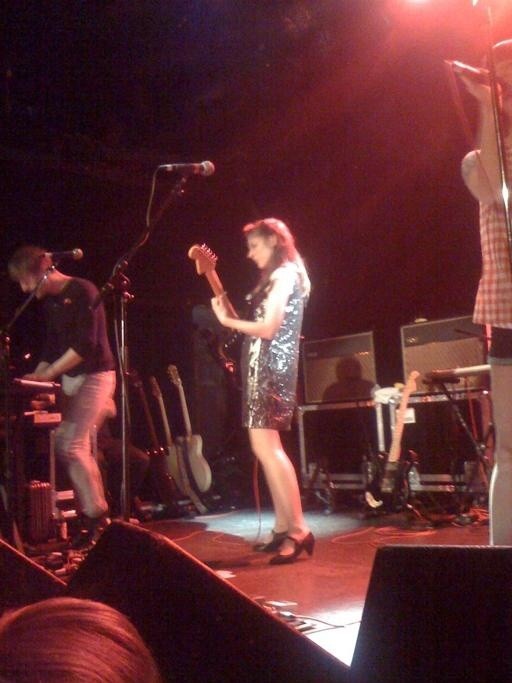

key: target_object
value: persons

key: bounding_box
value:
[96,422,149,522]
[0,596,162,683]
[211,218,313,562]
[461,40,512,547]
[8,244,116,542]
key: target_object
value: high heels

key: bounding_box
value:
[267,530,316,566]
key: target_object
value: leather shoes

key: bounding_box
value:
[252,529,291,553]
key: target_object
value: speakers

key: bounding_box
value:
[400,315,490,396]
[0,538,68,618]
[347,546,512,683]
[302,331,403,404]
[60,520,352,683]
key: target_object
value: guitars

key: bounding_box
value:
[167,365,213,496]
[148,375,191,494]
[190,244,242,383]
[127,369,177,505]
[365,371,423,513]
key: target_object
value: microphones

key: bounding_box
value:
[450,60,492,86]
[42,249,83,260]
[158,161,215,177]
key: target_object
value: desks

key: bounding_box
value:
[296,388,495,494]
